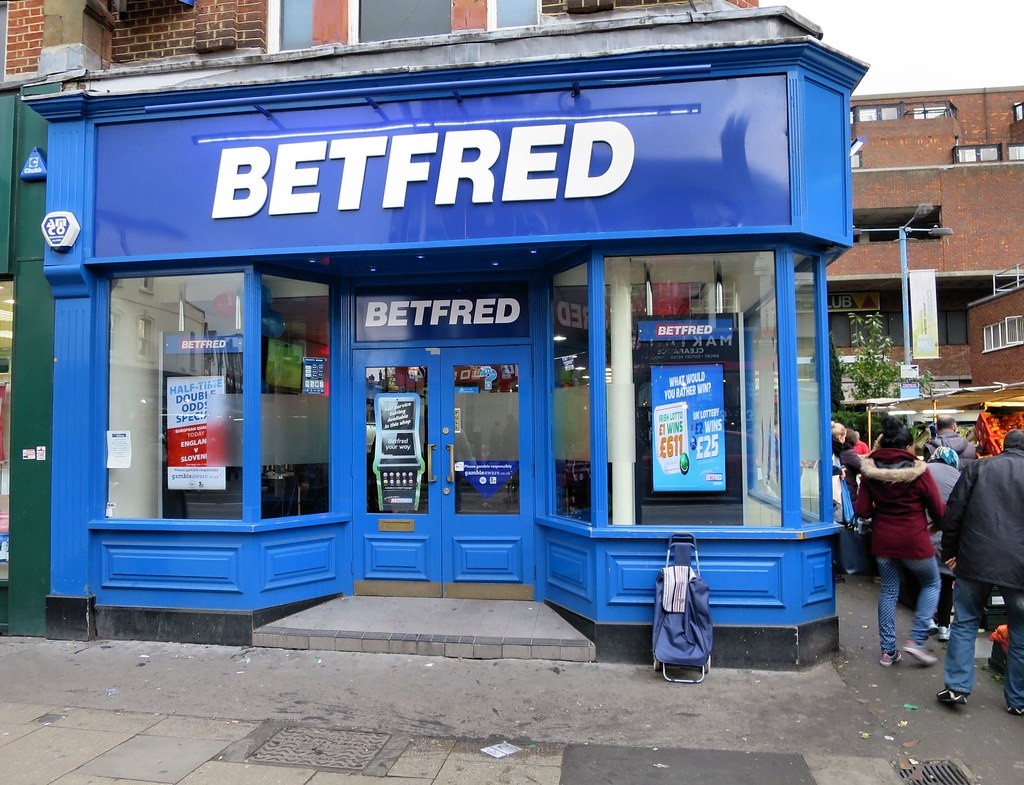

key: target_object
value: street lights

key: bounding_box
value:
[853,203,954,367]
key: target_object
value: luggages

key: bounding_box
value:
[651,543,713,670]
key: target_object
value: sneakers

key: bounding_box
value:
[937,624,958,640]
[928,619,939,636]
[904,640,941,666]
[881,650,903,666]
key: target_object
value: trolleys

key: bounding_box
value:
[650,531,713,683]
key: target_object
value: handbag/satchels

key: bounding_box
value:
[840,524,881,577]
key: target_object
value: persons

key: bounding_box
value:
[917,447,963,640]
[451,415,519,513]
[936,429,1024,716]
[922,418,976,471]
[854,415,949,667]
[830,422,872,582]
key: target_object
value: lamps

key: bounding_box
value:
[851,136,865,156]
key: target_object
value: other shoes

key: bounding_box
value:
[937,688,968,704]
[1007,705,1024,717]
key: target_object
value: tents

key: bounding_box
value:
[867,381,1024,454]
